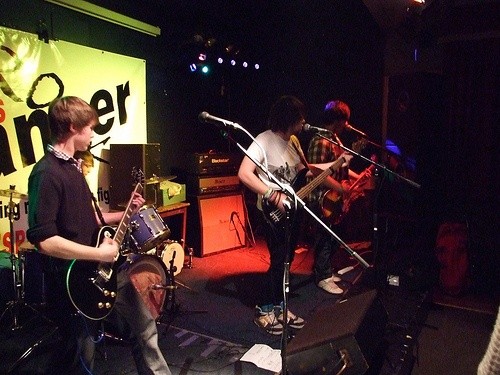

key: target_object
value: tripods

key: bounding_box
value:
[0,193,53,332]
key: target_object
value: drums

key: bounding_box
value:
[125,204,171,254]
[158,240,185,276]
[122,255,169,324]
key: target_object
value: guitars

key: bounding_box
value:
[261,137,368,230]
[321,153,378,225]
[66,168,145,321]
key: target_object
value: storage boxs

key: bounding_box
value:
[147,183,186,206]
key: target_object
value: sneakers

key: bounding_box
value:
[254,304,283,335]
[275,306,304,329]
[317,277,343,294]
[330,274,341,282]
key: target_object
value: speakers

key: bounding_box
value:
[283,286,390,375]
[109,143,158,212]
[189,190,248,258]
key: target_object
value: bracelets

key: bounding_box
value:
[264,188,281,207]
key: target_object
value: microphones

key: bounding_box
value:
[201,112,242,130]
[304,124,331,133]
[345,125,367,136]
[152,284,176,290]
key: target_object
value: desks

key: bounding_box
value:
[95,202,190,253]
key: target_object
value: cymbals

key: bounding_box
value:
[0,190,28,200]
[132,175,177,186]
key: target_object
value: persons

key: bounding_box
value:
[237,95,354,337]
[25,96,174,375]
[308,100,372,295]
[74,150,95,178]
